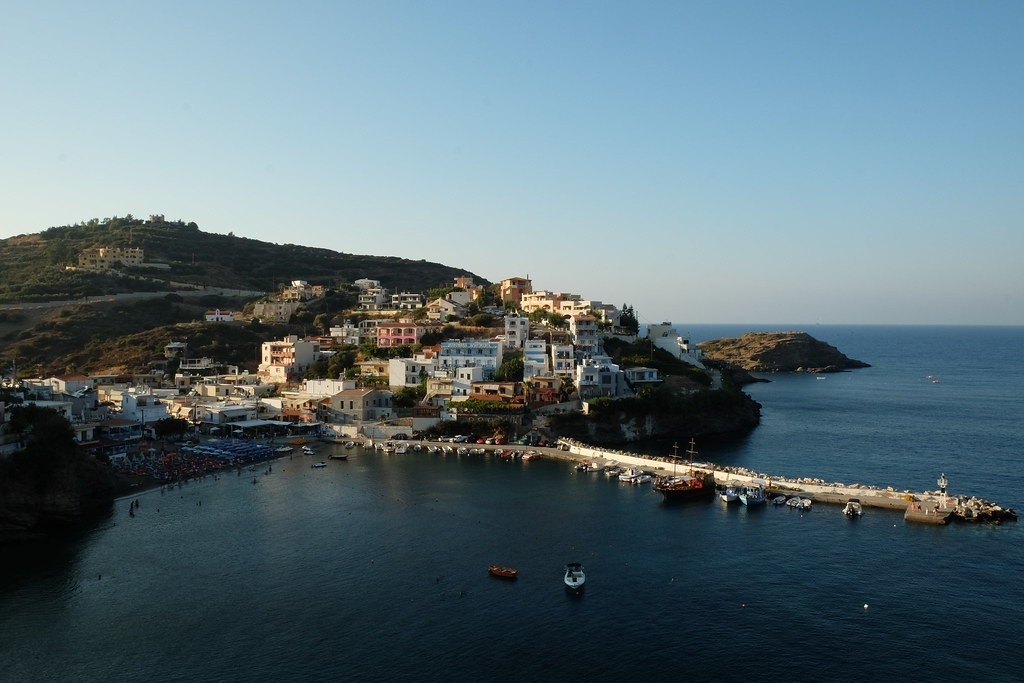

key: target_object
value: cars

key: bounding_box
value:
[518,439,529,445]
[533,440,556,448]
[477,436,506,445]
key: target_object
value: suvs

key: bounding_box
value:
[425,433,440,442]
[438,436,451,442]
[391,433,407,440]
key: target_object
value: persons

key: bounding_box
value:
[130,451,292,508]
[911,502,938,516]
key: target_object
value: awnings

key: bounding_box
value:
[220,418,320,440]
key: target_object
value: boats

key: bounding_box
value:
[604,461,651,484]
[786,496,811,510]
[720,485,737,502]
[312,462,327,468]
[652,438,716,502]
[302,446,316,455]
[773,495,786,504]
[738,480,767,506]
[364,438,373,449]
[328,454,348,460]
[842,498,862,515]
[374,441,409,454]
[427,445,441,454]
[494,449,539,460]
[469,448,486,455]
[442,445,453,454]
[345,441,355,449]
[413,444,421,452]
[574,461,604,472]
[457,447,468,455]
[488,564,519,577]
[564,563,585,589]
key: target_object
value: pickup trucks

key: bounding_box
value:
[449,435,468,443]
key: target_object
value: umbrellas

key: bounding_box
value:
[233,429,243,434]
[159,432,273,462]
[210,427,220,431]
